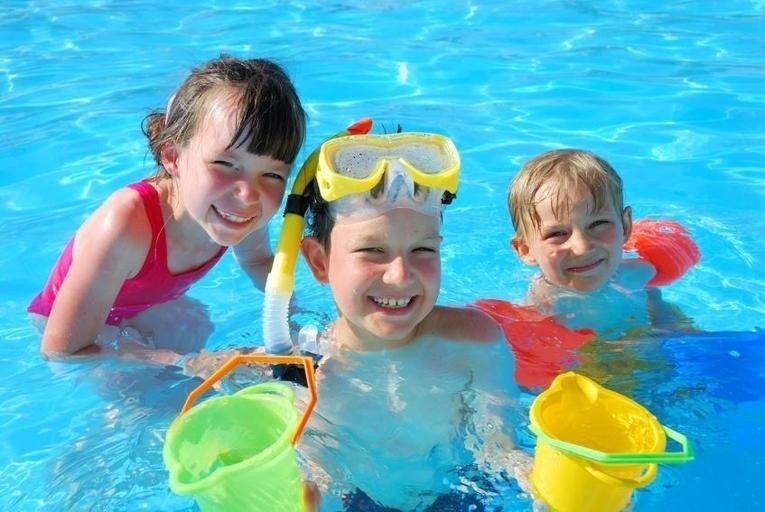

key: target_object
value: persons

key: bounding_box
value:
[299,121,551,512]
[507,149,658,339]
[27,57,305,394]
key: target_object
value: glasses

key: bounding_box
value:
[316,132,463,223]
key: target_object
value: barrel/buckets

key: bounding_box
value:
[161,350,318,512]
[527,369,697,512]
